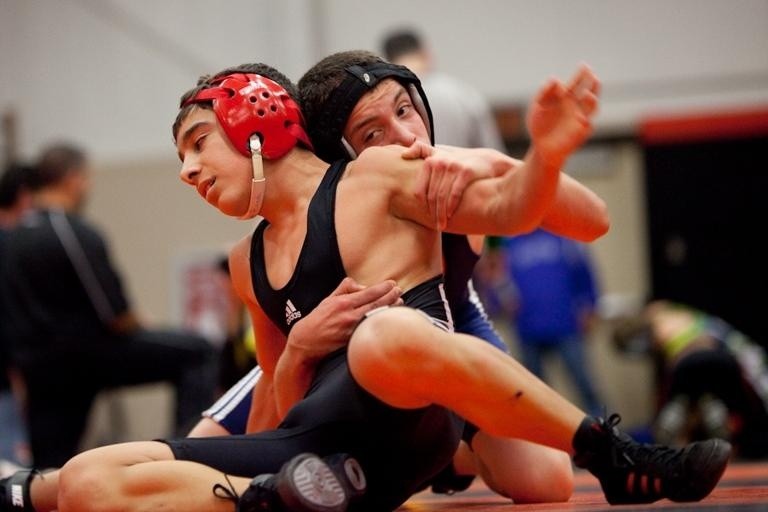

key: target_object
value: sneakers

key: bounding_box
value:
[233,452,368,512]
[577,417,732,506]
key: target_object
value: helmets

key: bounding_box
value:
[181,72,309,161]
[317,65,433,161]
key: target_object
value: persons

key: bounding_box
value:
[499,230,603,417]
[1,144,221,474]
[59,65,731,512]
[382,33,506,154]
[1,50,609,511]
[645,237,729,437]
